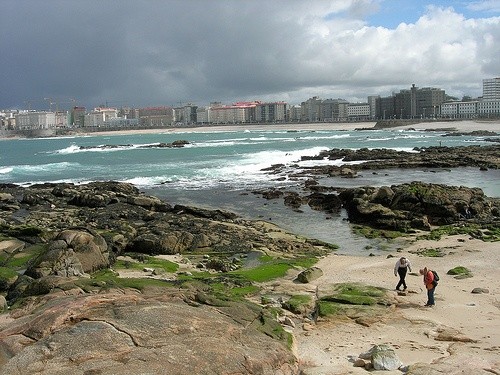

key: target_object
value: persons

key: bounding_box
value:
[419,266,440,306]
[394,257,412,290]
[439,141,442,146]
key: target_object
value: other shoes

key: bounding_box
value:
[424,302,435,307]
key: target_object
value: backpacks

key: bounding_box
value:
[427,270,439,281]
